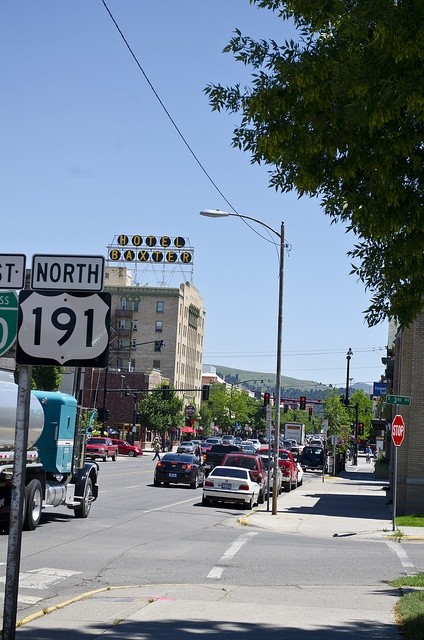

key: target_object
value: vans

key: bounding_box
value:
[301,446,326,472]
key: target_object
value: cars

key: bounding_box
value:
[191,434,260,453]
[202,466,262,510]
[113,439,143,457]
[257,448,299,491]
[154,452,205,488]
[310,439,323,446]
[177,440,196,453]
[221,453,269,503]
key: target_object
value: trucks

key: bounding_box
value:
[0,358,99,531]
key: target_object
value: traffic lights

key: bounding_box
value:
[300,397,306,409]
[358,423,364,435]
[264,392,270,405]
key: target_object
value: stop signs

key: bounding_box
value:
[392,415,405,447]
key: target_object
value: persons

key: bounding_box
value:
[151,440,160,461]
[365,445,372,461]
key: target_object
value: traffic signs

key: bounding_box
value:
[30,254,104,292]
[0,253,26,289]
[0,291,20,356]
[386,394,411,407]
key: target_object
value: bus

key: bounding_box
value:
[285,421,305,445]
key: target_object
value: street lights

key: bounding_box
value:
[199,209,286,514]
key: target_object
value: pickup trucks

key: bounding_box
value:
[203,444,242,472]
[85,437,119,461]
